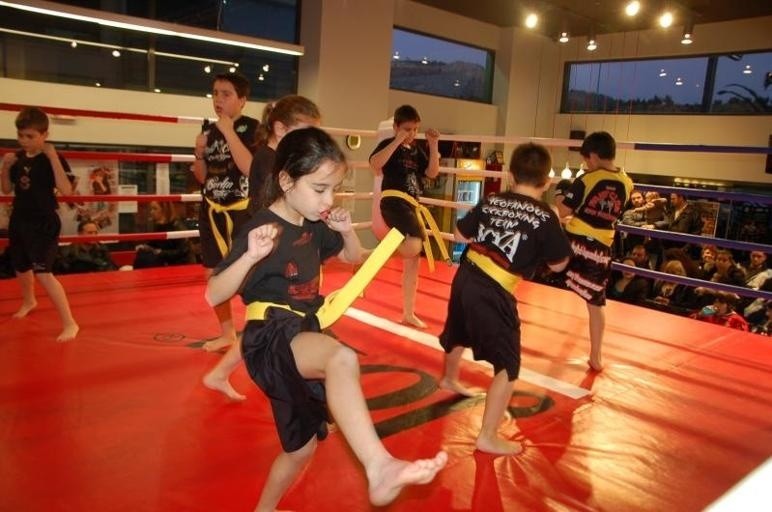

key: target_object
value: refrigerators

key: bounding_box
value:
[419,155,489,264]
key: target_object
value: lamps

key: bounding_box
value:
[625,0,704,44]
[518,0,608,51]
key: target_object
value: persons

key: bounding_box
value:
[204,127,448,512]
[244,95,337,434]
[354,103,440,328]
[1,201,200,282]
[555,131,634,372]
[604,190,772,337]
[437,142,573,454]
[193,74,262,352]
[1,108,79,343]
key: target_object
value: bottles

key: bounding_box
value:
[453,241,468,263]
[699,305,721,316]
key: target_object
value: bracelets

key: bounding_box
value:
[194,150,206,160]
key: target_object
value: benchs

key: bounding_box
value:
[110,249,139,271]
